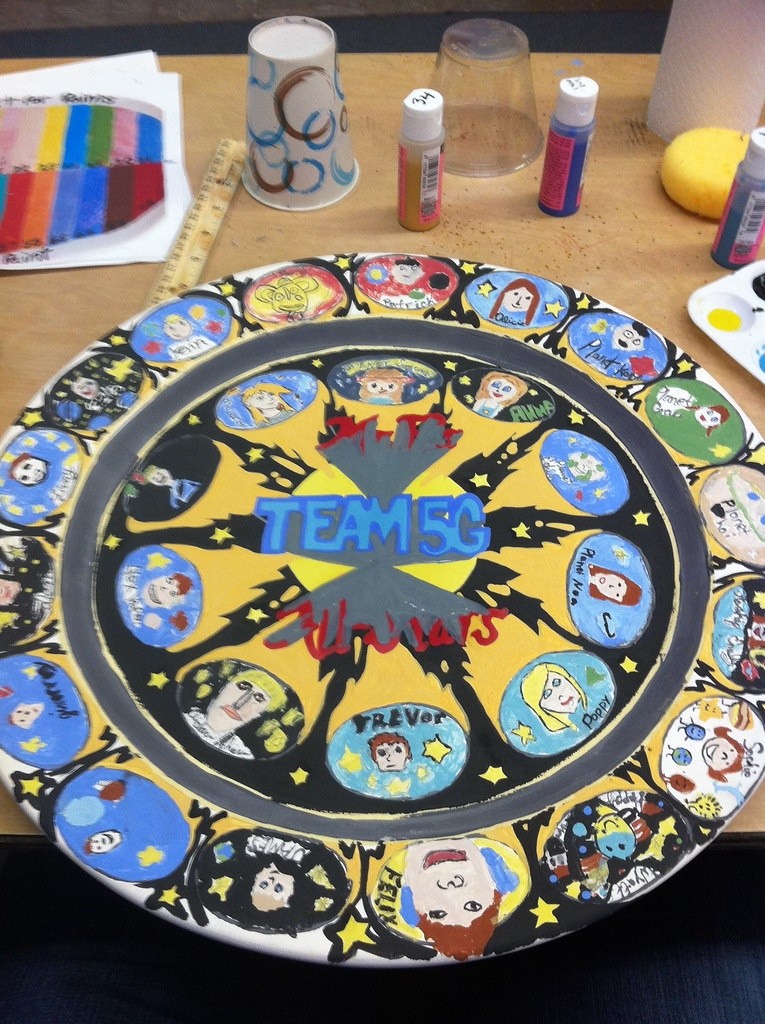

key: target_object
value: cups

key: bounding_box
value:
[240,12,360,210]
[430,21,539,186]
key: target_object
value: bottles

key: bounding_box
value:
[398,90,443,231]
[537,76,599,215]
[709,128,765,271]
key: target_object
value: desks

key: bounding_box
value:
[0,52,765,853]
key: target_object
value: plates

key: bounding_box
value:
[687,259,765,389]
